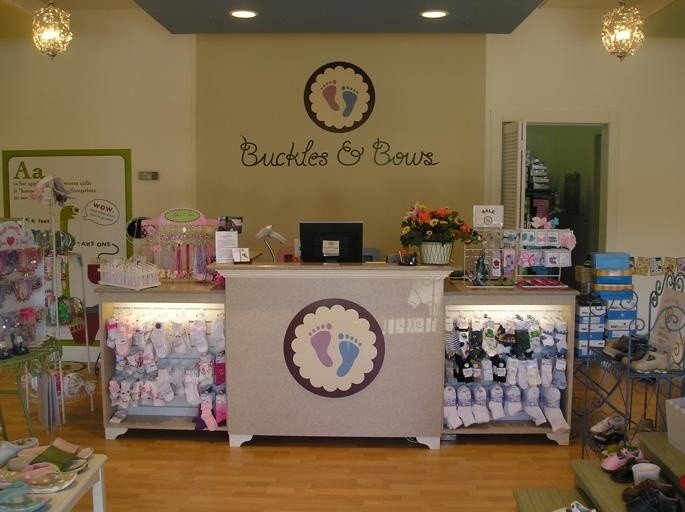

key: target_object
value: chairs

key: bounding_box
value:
[576,269,685,458]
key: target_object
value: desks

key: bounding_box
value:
[214,264,456,449]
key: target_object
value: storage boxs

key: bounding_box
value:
[575,340,606,358]
[581,281,592,295]
[592,283,633,300]
[666,398,685,453]
[605,318,636,339]
[592,269,633,284]
[574,265,593,283]
[606,299,638,320]
[593,252,630,270]
[575,305,607,324]
[576,323,604,340]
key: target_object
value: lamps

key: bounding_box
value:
[601,5,644,61]
[31,6,72,59]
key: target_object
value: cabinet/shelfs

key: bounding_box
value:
[447,279,580,446]
[94,280,226,440]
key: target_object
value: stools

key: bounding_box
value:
[511,486,600,512]
[636,431,685,495]
[569,455,685,512]
[0,449,110,512]
[44,339,100,364]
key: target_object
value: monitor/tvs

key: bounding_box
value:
[298,219,365,264]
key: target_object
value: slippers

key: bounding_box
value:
[8,447,88,473]
[1,482,51,512]
[17,437,95,459]
[1,436,38,470]
[0,462,78,495]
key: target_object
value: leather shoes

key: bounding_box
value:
[610,457,650,484]
[590,416,625,434]
[614,344,645,362]
[593,427,625,444]
[600,446,645,473]
[623,480,675,502]
[622,346,657,366]
[631,351,669,374]
[626,490,682,512]
[552,500,597,512]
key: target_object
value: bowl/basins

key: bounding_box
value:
[632,464,661,487]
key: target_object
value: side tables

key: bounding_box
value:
[0,335,63,437]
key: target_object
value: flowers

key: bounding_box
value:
[400,203,481,247]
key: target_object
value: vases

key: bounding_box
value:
[420,241,454,263]
